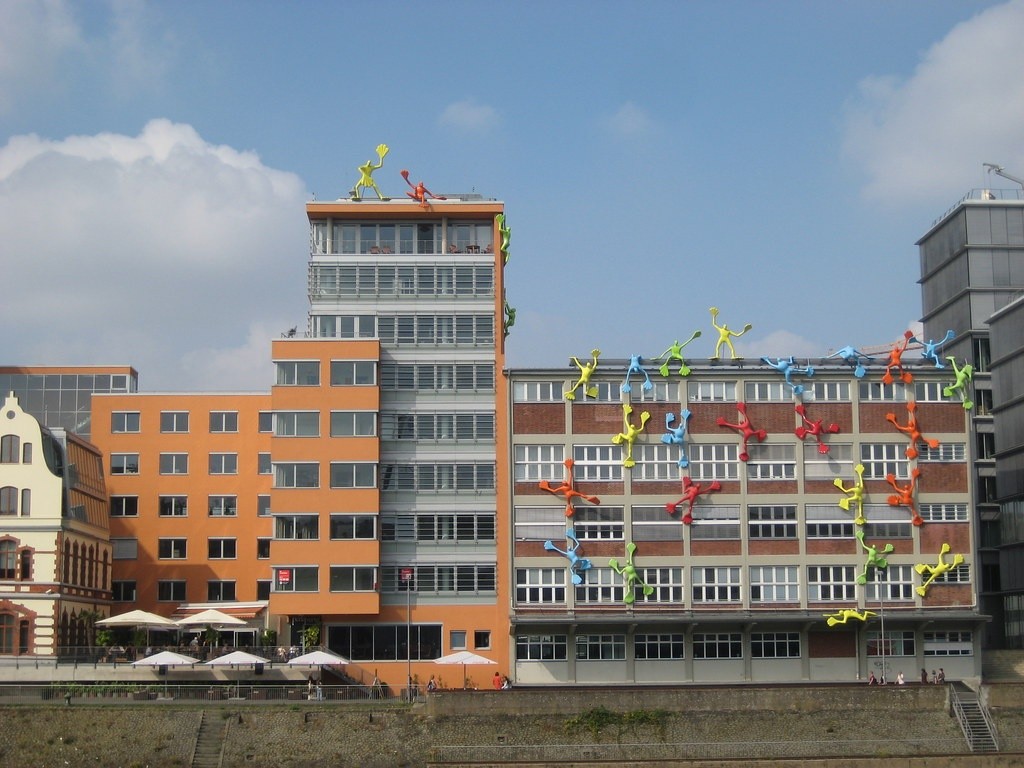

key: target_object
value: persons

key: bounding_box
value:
[307,672,322,700]
[882,331,913,385]
[493,672,509,690]
[109,637,230,656]
[886,402,938,460]
[716,402,767,462]
[943,356,974,409]
[650,330,701,377]
[539,459,600,517]
[820,346,874,378]
[856,531,894,585]
[621,354,652,393]
[428,675,436,690]
[400,170,446,207]
[914,543,964,596]
[564,349,601,400]
[794,404,838,454]
[412,685,418,702]
[834,465,866,525]
[886,468,923,525]
[496,214,510,262]
[609,543,654,604]
[868,668,945,687]
[351,144,390,200]
[665,477,721,525]
[708,308,752,360]
[612,405,651,468]
[761,356,814,395]
[544,528,592,584]
[909,330,955,369]
[661,410,691,468]
[504,304,517,336]
[277,644,299,661]
[823,610,877,627]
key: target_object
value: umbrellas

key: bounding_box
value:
[177,609,247,650]
[433,651,498,688]
[95,609,177,645]
[288,651,351,673]
[205,650,271,697]
[131,651,200,696]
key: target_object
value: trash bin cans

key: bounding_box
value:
[42,688,53,699]
[372,686,388,700]
[255,663,265,675]
[159,665,169,675]
